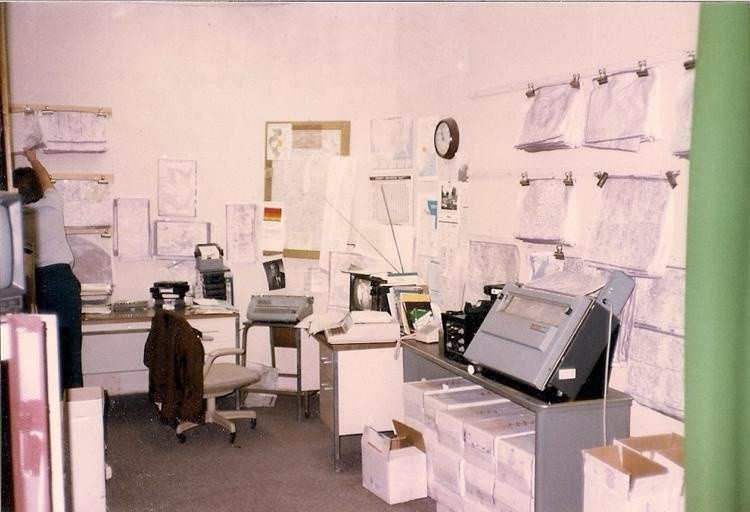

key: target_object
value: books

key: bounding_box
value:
[378,273,434,334]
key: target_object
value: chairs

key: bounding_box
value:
[152,310,256,444]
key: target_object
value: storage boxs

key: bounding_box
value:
[612,432,685,465]
[580,445,670,512]
[403,377,536,512]
[361,419,428,506]
[650,445,685,512]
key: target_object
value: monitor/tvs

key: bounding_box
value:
[0,191,27,314]
[350,274,392,317]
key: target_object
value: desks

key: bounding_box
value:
[241,319,403,473]
[400,339,634,512]
[81,313,240,410]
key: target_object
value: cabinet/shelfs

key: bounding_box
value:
[685,1,750,512]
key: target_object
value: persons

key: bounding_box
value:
[11,145,84,400]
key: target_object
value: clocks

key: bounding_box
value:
[433,117,459,159]
